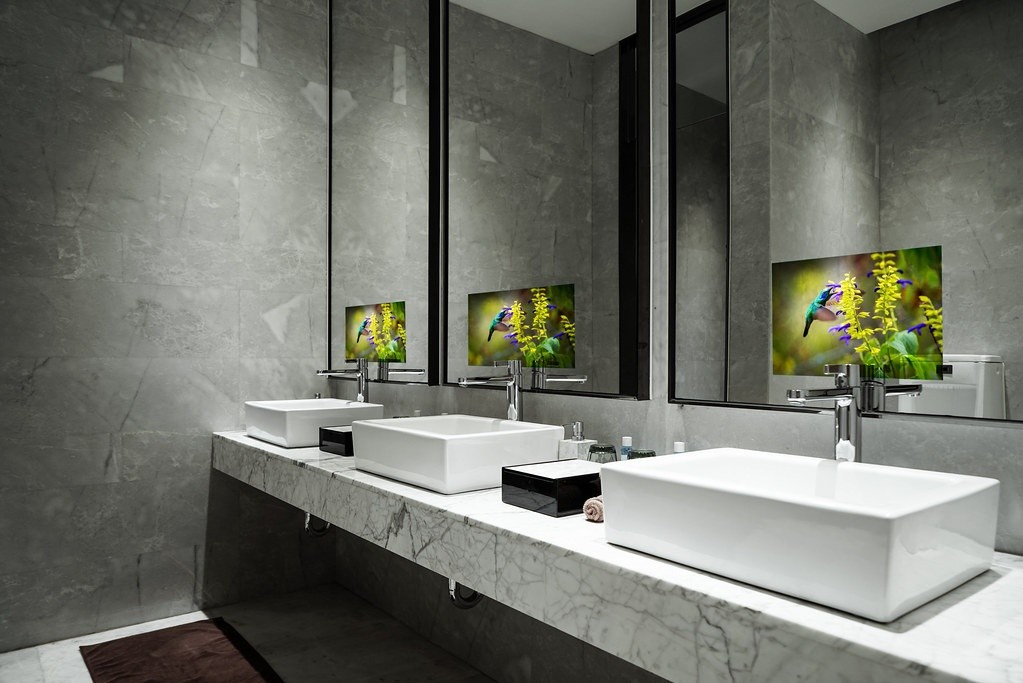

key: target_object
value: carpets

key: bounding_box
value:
[79,614,287,683]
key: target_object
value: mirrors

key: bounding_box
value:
[667,0,1023,422]
[327,0,440,388]
[441,0,652,403]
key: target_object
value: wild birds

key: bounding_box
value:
[487,309,509,342]
[356,319,369,343]
[802,286,837,337]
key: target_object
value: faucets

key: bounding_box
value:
[785,363,868,464]
[457,359,524,421]
[530,359,588,390]
[859,363,923,415]
[316,357,369,404]
[376,358,426,381]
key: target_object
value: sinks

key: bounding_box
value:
[351,414,566,496]
[243,397,384,449]
[598,446,1001,624]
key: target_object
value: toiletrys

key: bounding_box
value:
[619,436,633,461]
[557,420,599,460]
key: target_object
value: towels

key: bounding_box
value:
[582,494,603,522]
